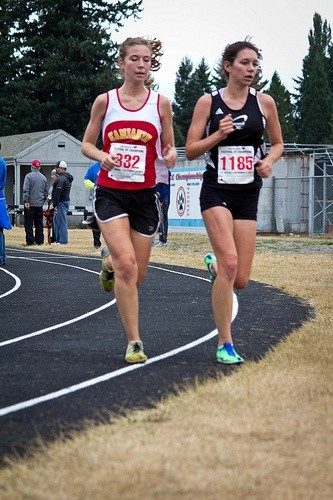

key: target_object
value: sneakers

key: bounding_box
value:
[204,253,216,284]
[50,242,69,246]
[126,340,149,364]
[100,253,117,295]
[215,343,245,364]
[156,241,167,247]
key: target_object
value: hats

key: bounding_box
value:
[50,169,59,177]
[55,160,68,169]
[30,160,41,169]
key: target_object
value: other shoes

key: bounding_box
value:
[0,260,5,266]
[21,242,29,246]
[94,245,99,250]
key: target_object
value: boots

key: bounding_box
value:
[93,229,101,248]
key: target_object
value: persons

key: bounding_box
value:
[0,143,12,266]
[185,41,285,365]
[23,160,72,246]
[154,170,172,247]
[83,150,104,250]
[80,36,176,364]
